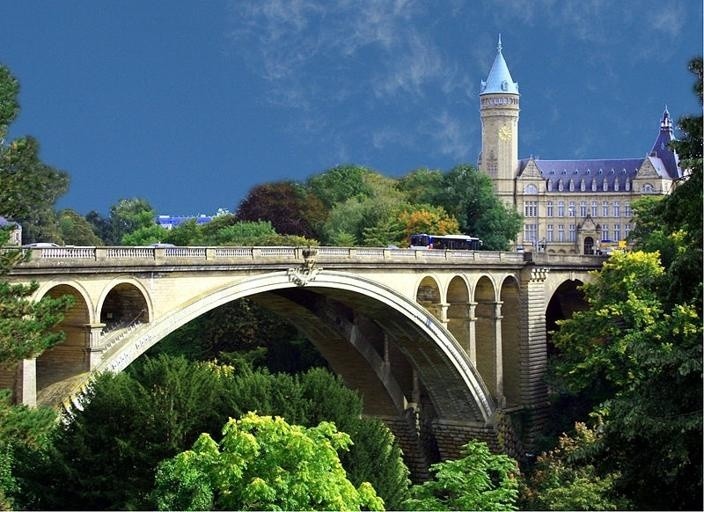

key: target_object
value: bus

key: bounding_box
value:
[409,232,484,250]
